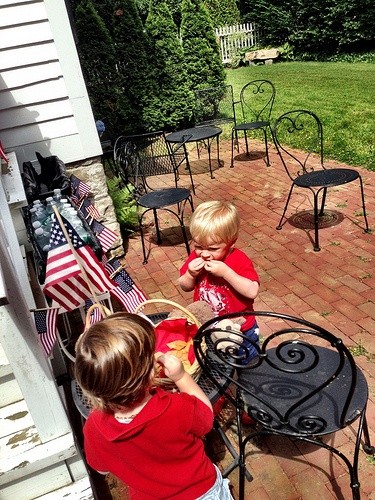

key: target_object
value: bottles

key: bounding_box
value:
[29,188,89,253]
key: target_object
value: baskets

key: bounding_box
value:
[73,304,111,406]
[134,299,207,391]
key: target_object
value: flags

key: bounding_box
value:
[90,219,119,254]
[80,199,100,220]
[69,195,81,209]
[44,212,119,313]
[85,216,92,224]
[104,258,120,276]
[110,269,149,314]
[71,176,90,198]
[34,308,59,356]
[84,299,102,325]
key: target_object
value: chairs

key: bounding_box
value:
[125,131,195,216]
[230,80,276,168]
[273,110,372,252]
[193,311,375,500]
[185,86,240,158]
[114,135,195,265]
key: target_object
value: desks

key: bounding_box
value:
[70,312,253,482]
[166,127,224,179]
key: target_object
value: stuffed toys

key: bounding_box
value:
[209,316,248,354]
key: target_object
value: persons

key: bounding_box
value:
[177,200,262,427]
[74,311,235,500]
